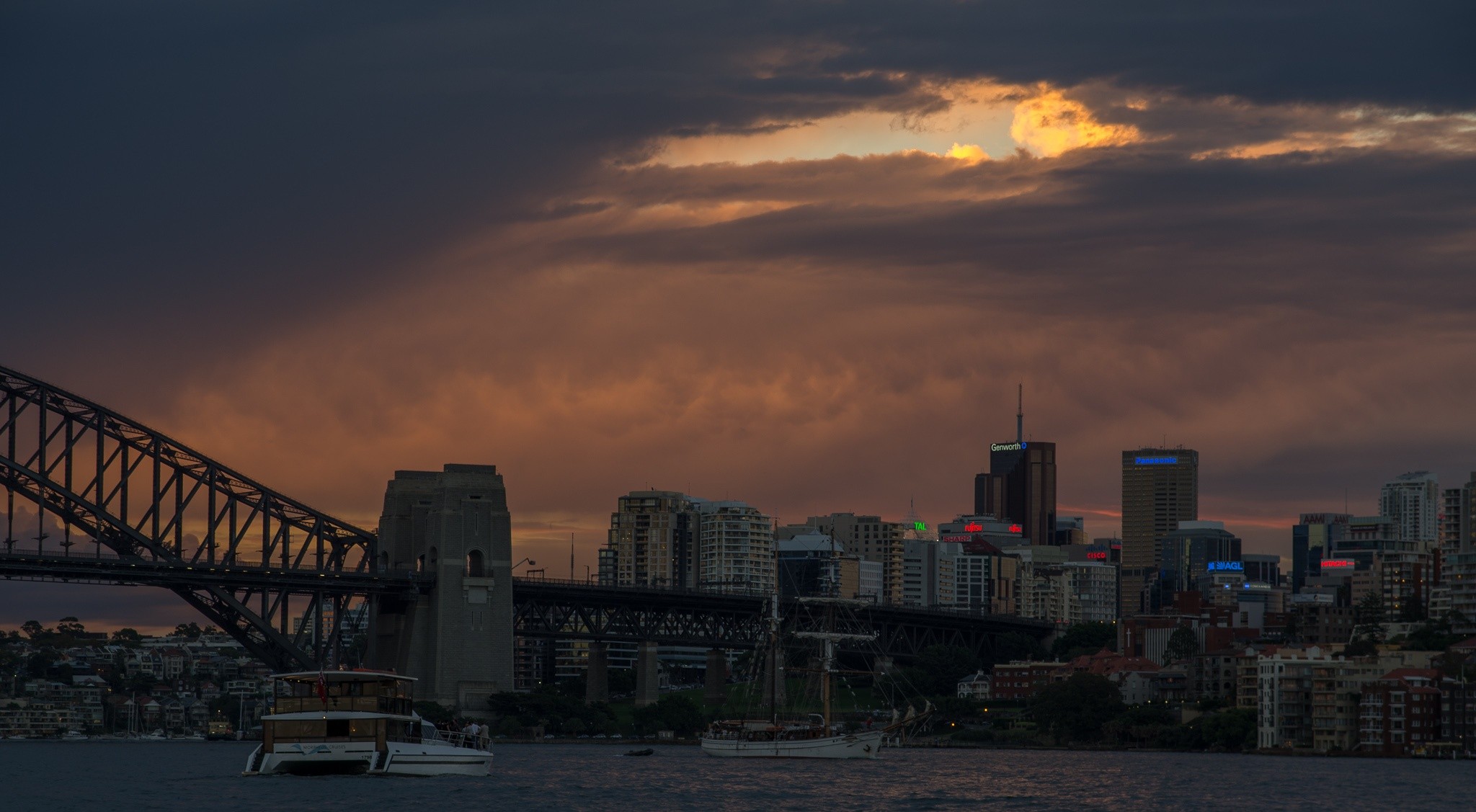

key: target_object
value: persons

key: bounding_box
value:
[702,728,740,740]
[436,719,490,751]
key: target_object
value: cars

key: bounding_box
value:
[496,734,506,738]
[578,734,589,739]
[610,733,622,739]
[544,734,555,740]
[593,733,606,740]
[643,733,655,739]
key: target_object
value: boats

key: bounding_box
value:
[204,711,237,741]
[8,735,25,740]
[62,730,87,740]
[246,669,495,780]
[624,748,654,757]
[148,729,204,740]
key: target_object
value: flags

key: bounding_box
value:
[317,671,326,704]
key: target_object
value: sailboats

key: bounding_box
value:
[98,690,150,740]
[694,523,938,760]
